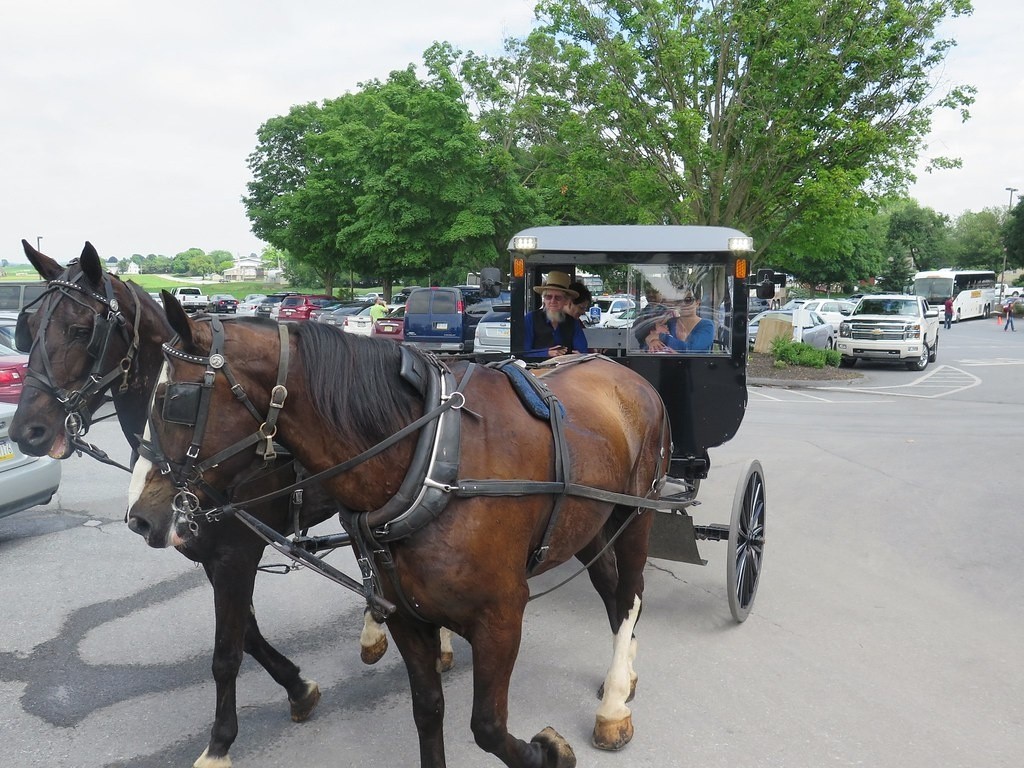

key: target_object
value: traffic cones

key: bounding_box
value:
[997,316,1004,326]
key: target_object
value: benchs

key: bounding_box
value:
[583,328,640,357]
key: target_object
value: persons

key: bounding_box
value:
[1005,302,1017,332]
[370,296,389,323]
[943,297,953,330]
[560,281,597,354]
[632,281,677,349]
[524,270,588,361]
[646,283,714,353]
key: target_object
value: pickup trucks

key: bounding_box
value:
[995,284,1024,297]
[170,286,209,314]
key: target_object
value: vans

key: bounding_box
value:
[148,293,163,309]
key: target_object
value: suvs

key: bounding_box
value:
[836,294,939,372]
[236,285,512,364]
[0,280,50,313]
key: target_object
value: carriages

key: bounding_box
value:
[8,224,775,762]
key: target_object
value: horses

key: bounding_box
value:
[9,238,673,768]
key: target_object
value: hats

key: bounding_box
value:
[533,270,579,301]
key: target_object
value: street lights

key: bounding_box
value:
[36,236,43,282]
[1004,186,1018,212]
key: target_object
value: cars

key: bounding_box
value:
[209,294,239,314]
[1002,297,1024,312]
[0,344,62,519]
[579,294,649,330]
[747,295,861,361]
[0,311,31,356]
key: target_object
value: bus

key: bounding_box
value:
[914,269,996,323]
[749,270,788,311]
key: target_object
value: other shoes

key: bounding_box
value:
[1004,329,1007,331]
[1013,329,1016,331]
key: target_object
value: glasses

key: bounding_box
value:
[543,294,566,301]
[645,289,660,294]
[674,296,697,305]
